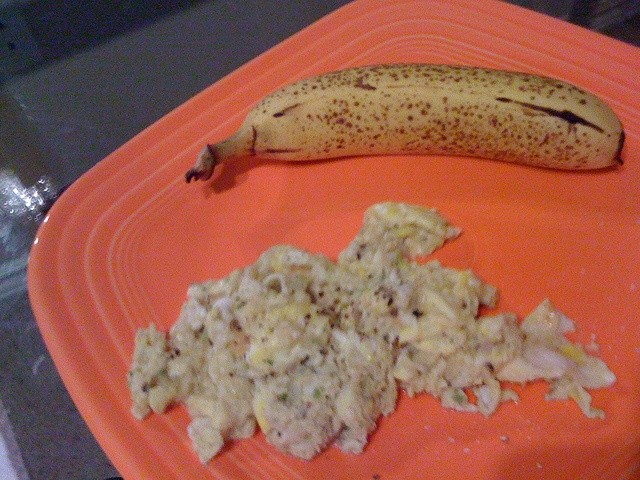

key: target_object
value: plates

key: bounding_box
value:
[29,0,638,479]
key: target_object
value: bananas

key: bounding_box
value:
[183,63,625,185]
